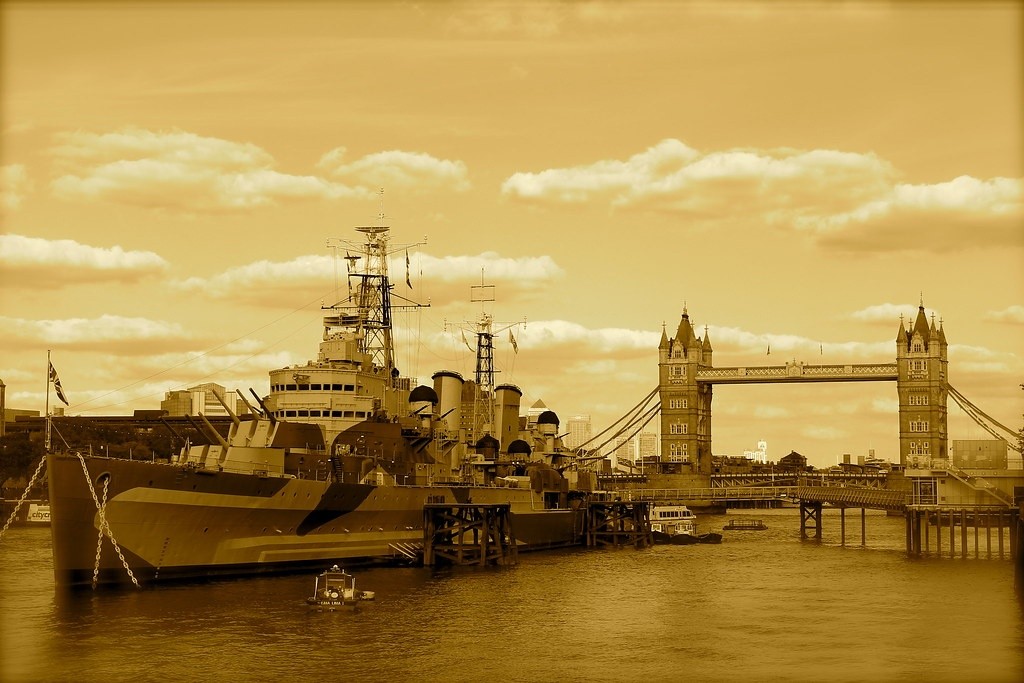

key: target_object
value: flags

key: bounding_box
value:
[49,361,69,407]
[462,331,475,352]
[405,250,413,290]
[508,330,519,354]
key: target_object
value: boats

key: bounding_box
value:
[306,564,358,612]
[670,520,698,545]
[45,187,604,588]
[697,527,722,543]
[644,504,697,526]
[646,523,670,544]
